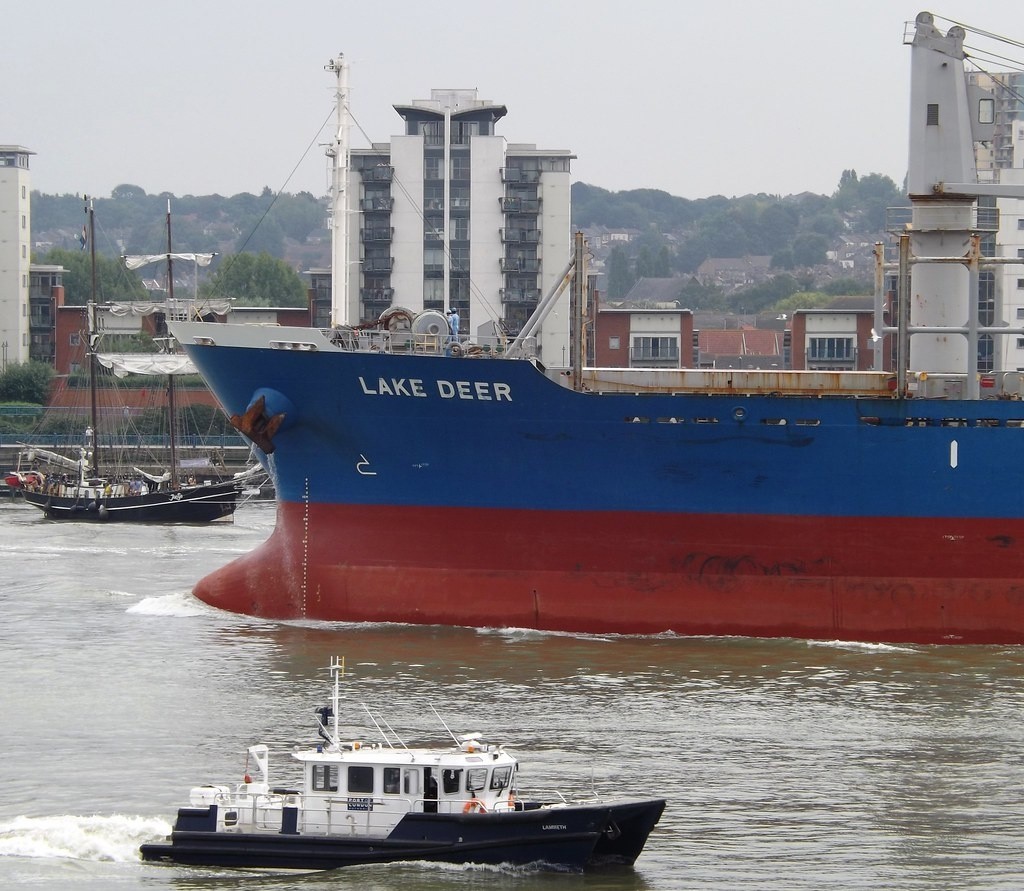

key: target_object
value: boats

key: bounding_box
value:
[141,653,667,871]
[159,51,1024,648]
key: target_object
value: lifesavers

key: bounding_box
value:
[463,798,486,813]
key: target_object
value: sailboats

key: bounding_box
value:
[5,199,240,524]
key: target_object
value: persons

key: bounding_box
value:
[445,307,460,344]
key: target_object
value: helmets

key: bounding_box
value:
[451,307,457,312]
[447,310,452,314]
